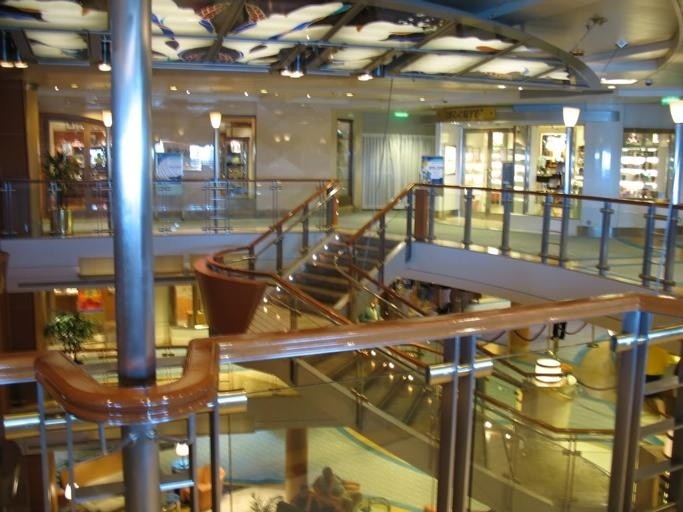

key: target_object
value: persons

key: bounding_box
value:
[248,466,362,512]
[166,489,180,508]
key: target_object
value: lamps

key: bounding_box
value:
[357,69,373,80]
[0,48,14,69]
[291,50,305,79]
[96,40,113,72]
[279,57,291,77]
[14,46,30,70]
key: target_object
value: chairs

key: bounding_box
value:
[179,465,226,512]
